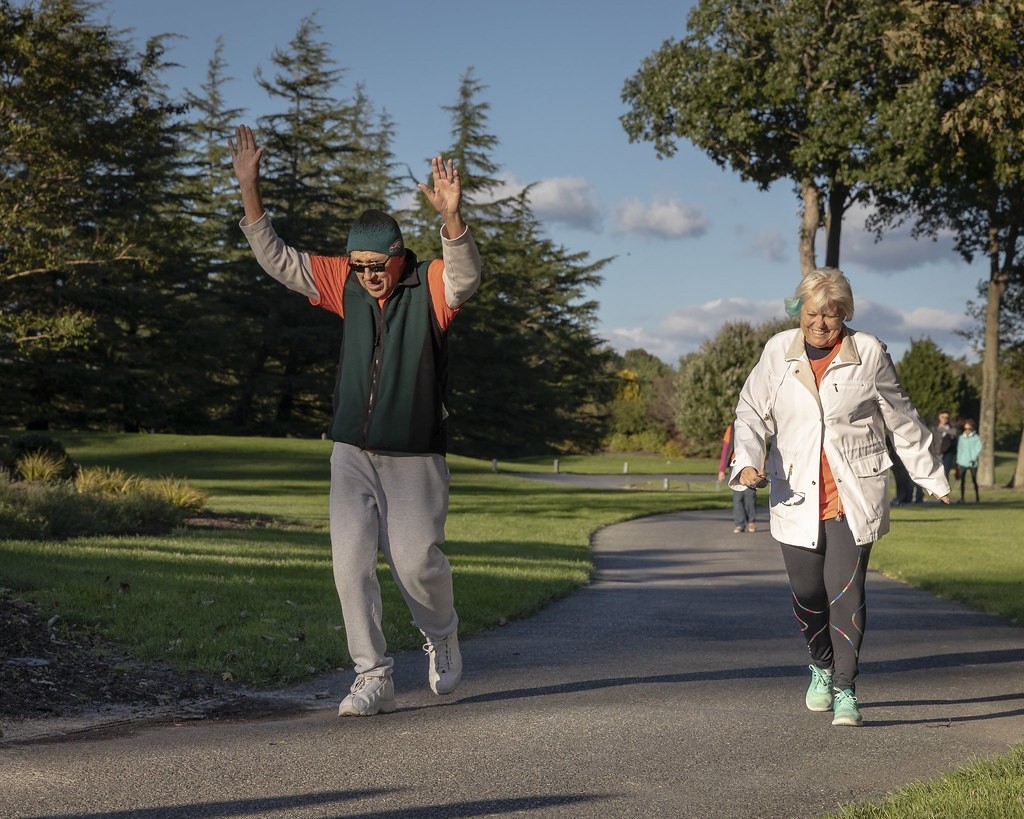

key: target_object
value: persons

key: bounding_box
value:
[230,126,487,720]
[717,421,759,535]
[883,425,926,506]
[955,419,983,503]
[928,410,959,504]
[726,267,955,728]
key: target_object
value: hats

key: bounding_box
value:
[347,209,405,256]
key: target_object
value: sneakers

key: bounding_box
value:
[339,668,396,716]
[806,661,834,711]
[419,629,463,694]
[832,687,862,725]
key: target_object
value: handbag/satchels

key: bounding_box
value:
[956,464,960,480]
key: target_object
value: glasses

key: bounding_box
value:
[964,428,972,430]
[348,255,391,272]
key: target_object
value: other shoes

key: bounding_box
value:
[890,498,923,507]
[747,523,756,532]
[733,526,745,532]
[957,499,966,504]
